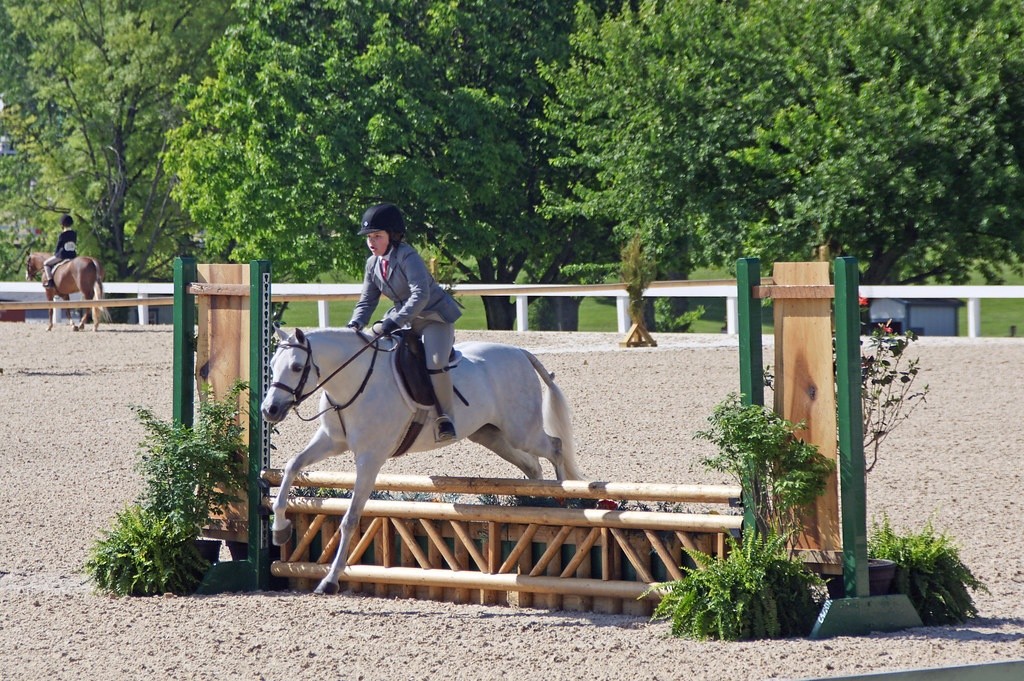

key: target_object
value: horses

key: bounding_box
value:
[261,322,584,595]
[25,251,112,332]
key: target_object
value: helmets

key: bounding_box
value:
[357,203,406,243]
[59,215,73,225]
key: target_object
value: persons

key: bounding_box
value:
[42,216,77,286]
[344,204,462,440]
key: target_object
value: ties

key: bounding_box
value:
[381,259,389,281]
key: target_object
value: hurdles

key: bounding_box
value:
[171,255,927,639]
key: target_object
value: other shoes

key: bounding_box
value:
[437,422,456,440]
[43,279,54,288]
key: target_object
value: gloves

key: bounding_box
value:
[346,321,362,331]
[380,318,401,337]
